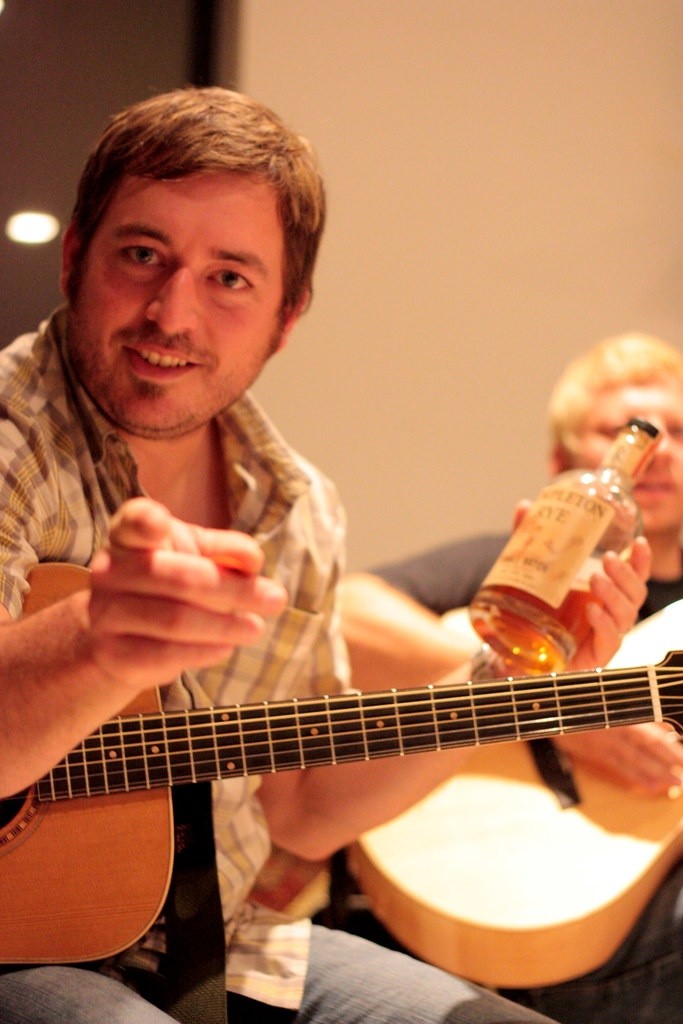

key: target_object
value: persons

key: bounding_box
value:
[0,86,652,1024]
[335,332,683,801]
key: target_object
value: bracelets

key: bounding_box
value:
[470,644,497,682]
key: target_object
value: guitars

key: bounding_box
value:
[0,562,683,965]
[344,598,683,989]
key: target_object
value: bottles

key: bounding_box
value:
[470,415,664,678]
